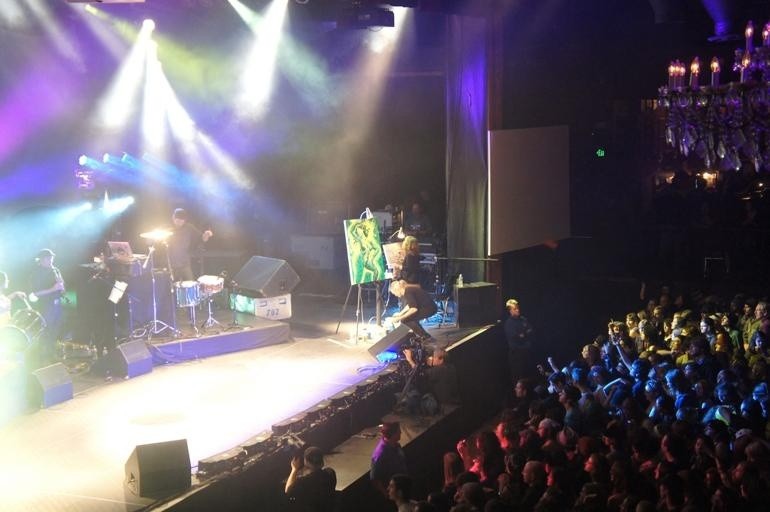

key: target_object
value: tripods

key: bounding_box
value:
[183,289,250,335]
[144,257,182,338]
[334,217,389,343]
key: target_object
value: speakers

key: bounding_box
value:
[122,437,192,497]
[30,360,73,408]
[110,336,153,379]
[367,322,419,359]
[226,254,303,298]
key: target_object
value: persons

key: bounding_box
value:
[148,204,214,282]
[29,248,67,346]
[365,416,411,512]
[386,278,770,512]
[0,270,27,319]
[281,445,338,512]
[347,219,380,285]
[391,235,426,285]
[404,203,435,236]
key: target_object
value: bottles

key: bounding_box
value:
[433,274,441,296]
[91,345,108,361]
[457,273,464,288]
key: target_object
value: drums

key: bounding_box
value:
[196,275,224,293]
[2,309,46,355]
[172,281,199,308]
